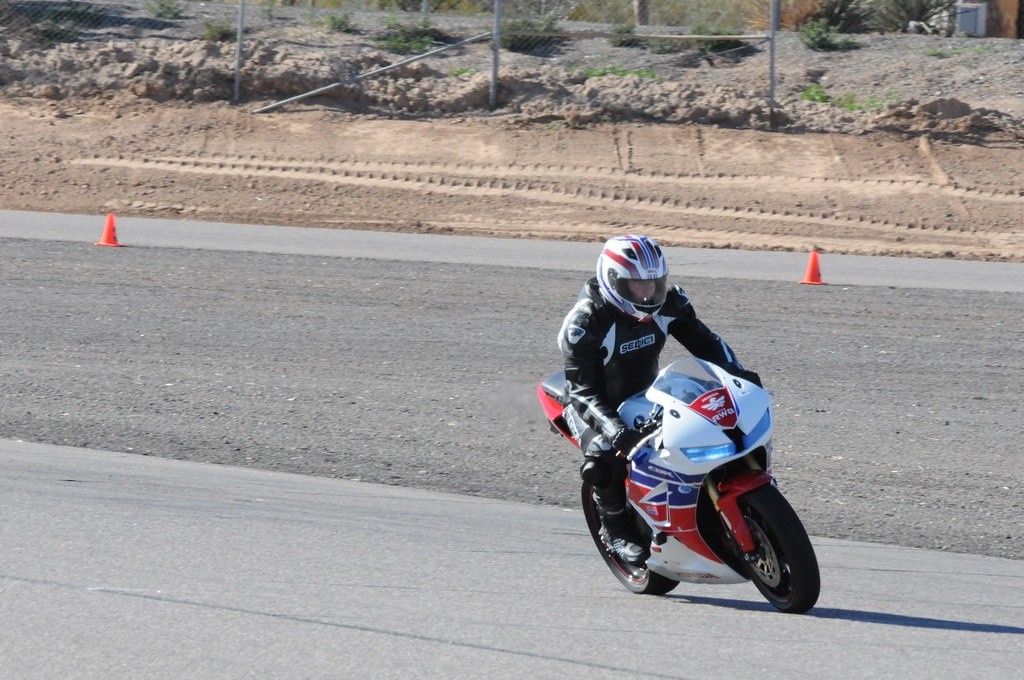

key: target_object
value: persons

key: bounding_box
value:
[557,233,762,563]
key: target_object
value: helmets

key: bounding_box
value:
[596,235,669,323]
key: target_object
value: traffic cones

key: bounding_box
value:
[800,252,827,284]
[94,214,124,246]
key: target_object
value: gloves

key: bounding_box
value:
[612,428,639,456]
[743,370,763,388]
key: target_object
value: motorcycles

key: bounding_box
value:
[536,356,820,615]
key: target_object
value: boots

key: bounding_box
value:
[593,485,648,563]
